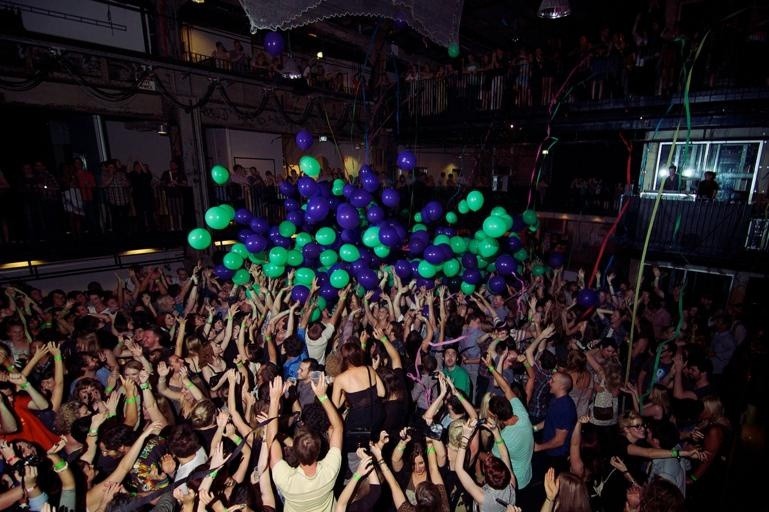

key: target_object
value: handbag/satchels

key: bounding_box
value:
[343,425,374,455]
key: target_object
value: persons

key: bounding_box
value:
[2,17,769,512]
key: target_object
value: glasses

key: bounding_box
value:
[625,423,649,430]
